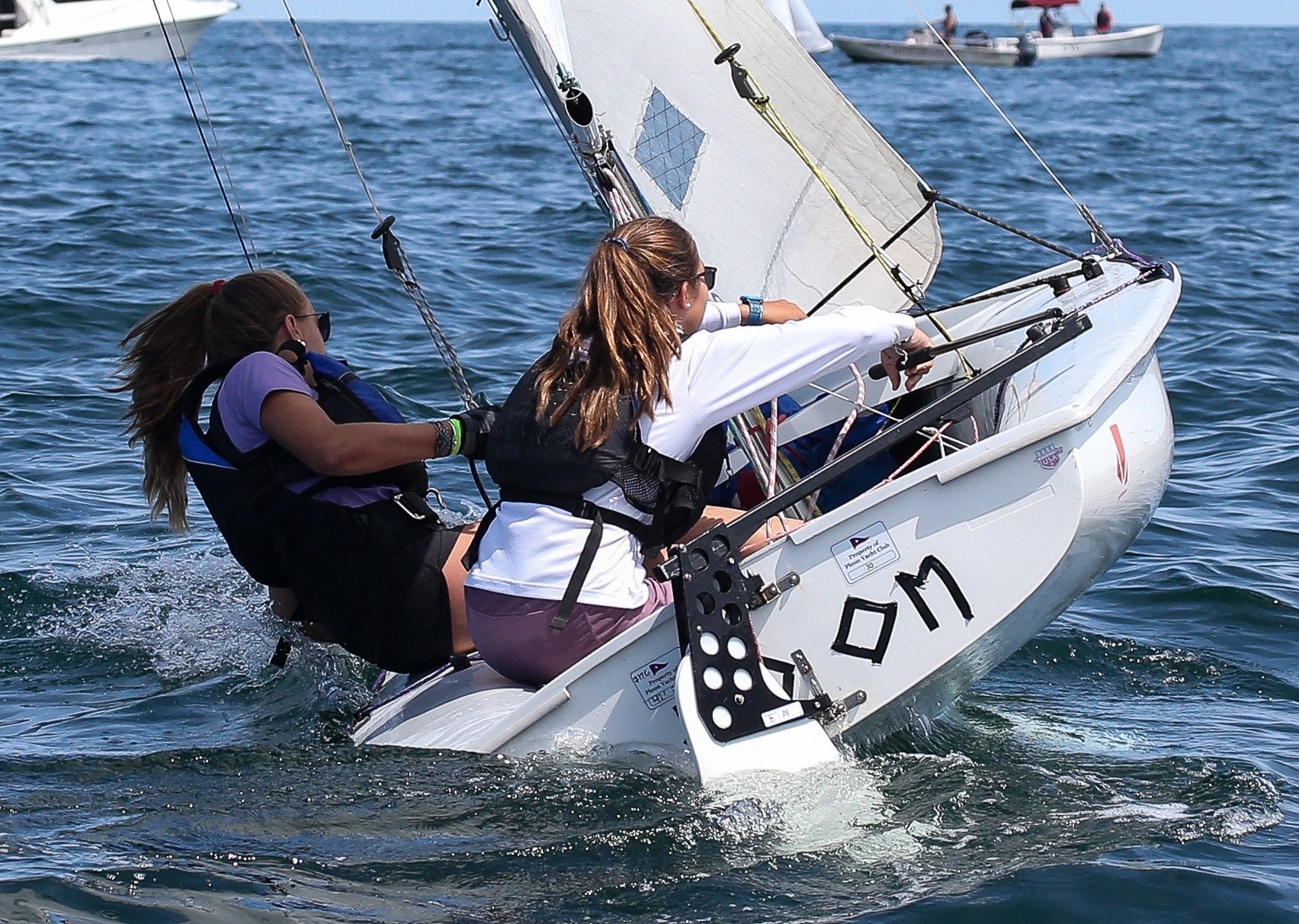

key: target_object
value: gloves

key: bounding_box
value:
[449,401,504,461]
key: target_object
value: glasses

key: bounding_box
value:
[294,311,332,343]
[690,266,717,291]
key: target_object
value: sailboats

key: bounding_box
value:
[155,0,1186,791]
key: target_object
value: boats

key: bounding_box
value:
[826,0,1168,66]
[1,1,240,64]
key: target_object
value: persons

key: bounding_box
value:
[125,269,503,669]
[933,4,957,45]
[1096,3,1113,34]
[462,214,937,686]
[1041,4,1059,38]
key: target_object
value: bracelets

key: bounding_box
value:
[740,295,765,326]
[426,418,463,459]
